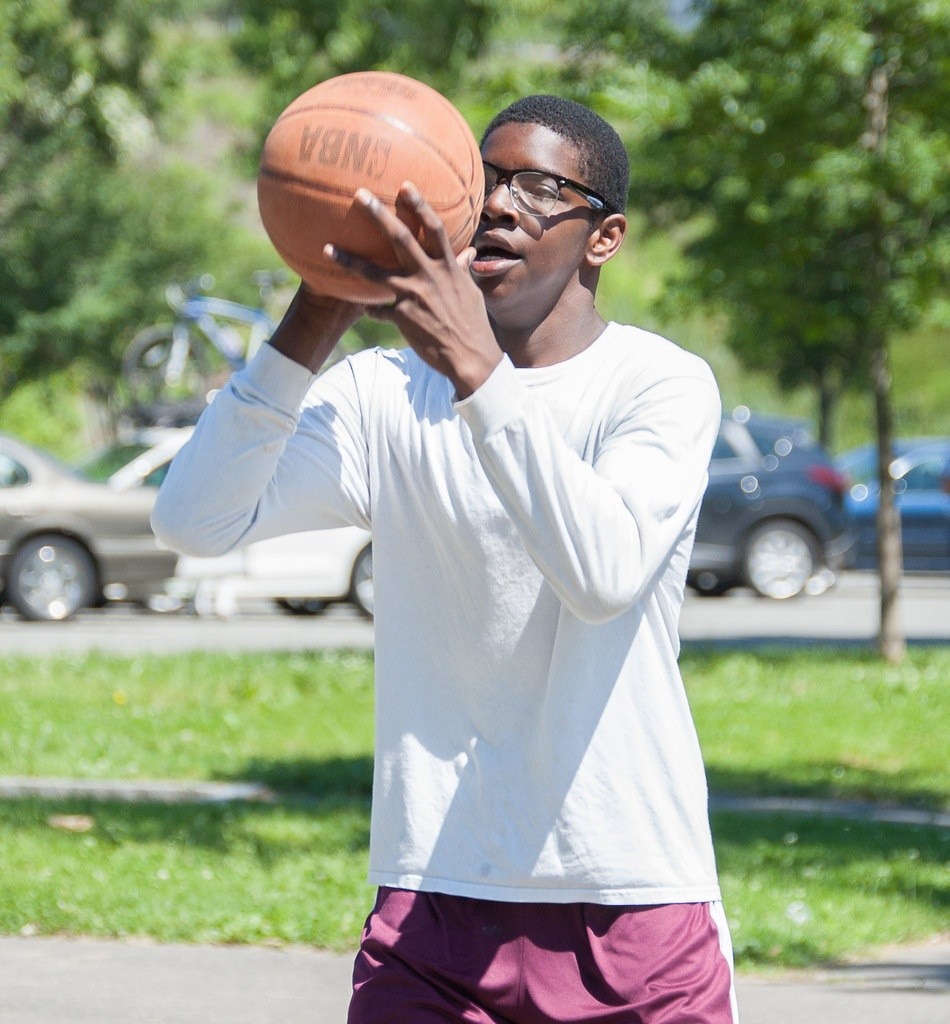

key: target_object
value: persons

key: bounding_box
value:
[152,91,742,1024]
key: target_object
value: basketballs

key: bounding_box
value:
[258,70,487,307]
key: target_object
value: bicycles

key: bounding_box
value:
[121,261,353,429]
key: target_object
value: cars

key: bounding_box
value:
[831,434,950,581]
[685,411,849,599]
[0,437,178,631]
[69,433,383,625]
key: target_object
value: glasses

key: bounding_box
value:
[482,159,618,218]
